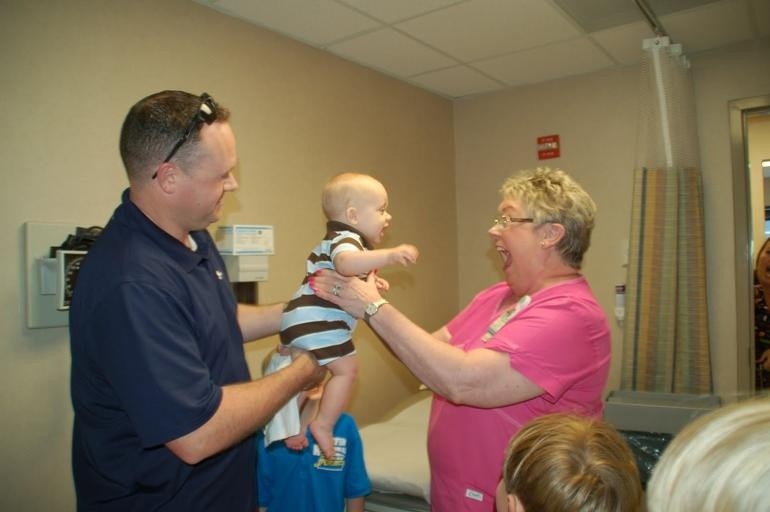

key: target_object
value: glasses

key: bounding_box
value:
[152,91,217,180]
[494,215,545,231]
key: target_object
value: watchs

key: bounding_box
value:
[364,299,390,325]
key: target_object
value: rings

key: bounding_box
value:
[332,288,340,296]
[334,281,342,289]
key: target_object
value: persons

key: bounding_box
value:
[754,237,770,390]
[70,89,327,512]
[279,171,420,461]
[308,166,610,511]
[256,349,373,512]
[495,396,770,512]
